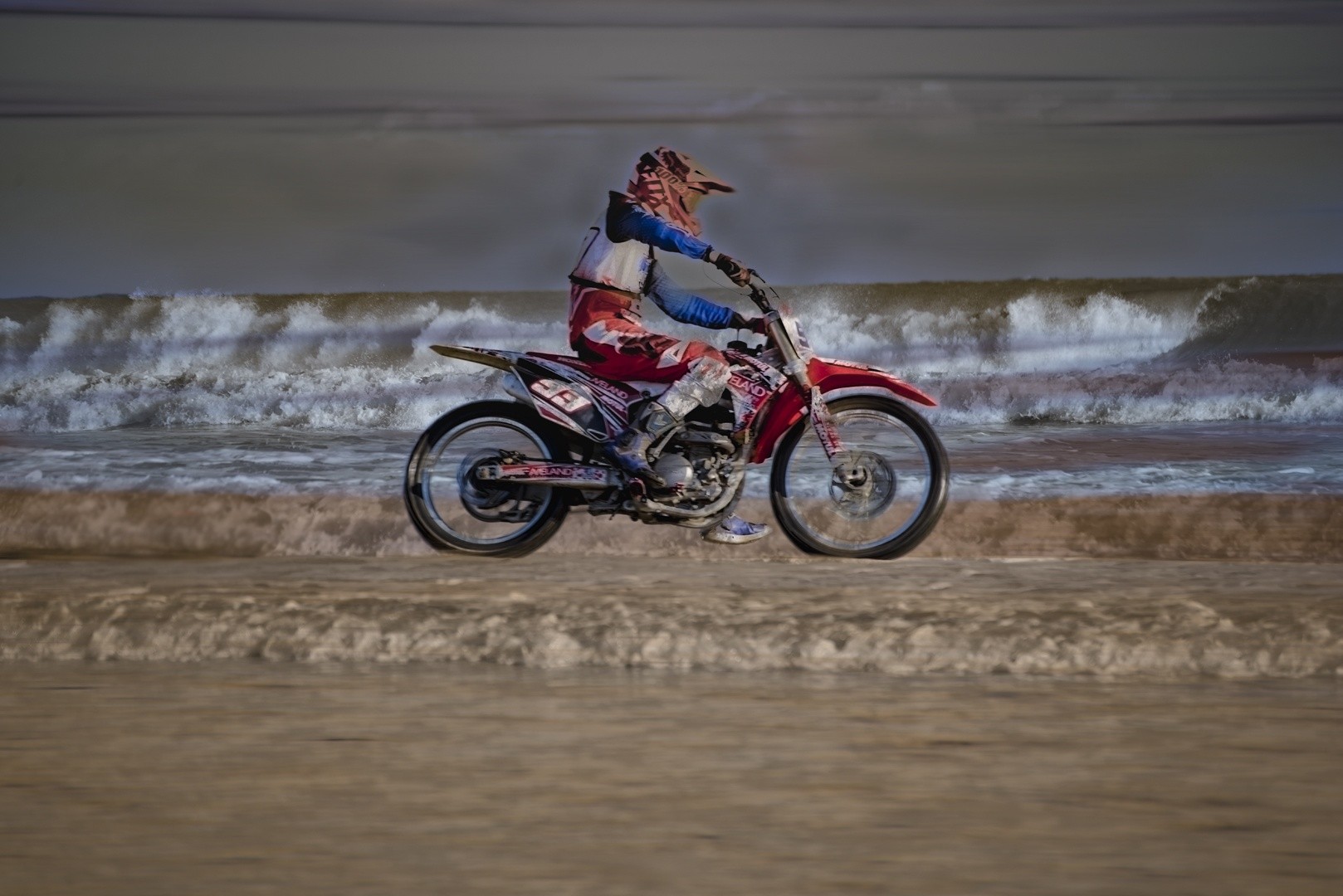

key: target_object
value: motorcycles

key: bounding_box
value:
[403,281,952,560]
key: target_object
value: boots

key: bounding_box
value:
[701,512,772,544]
[600,400,678,487]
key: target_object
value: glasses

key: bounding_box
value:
[682,187,703,213]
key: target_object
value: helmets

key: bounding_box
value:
[628,147,735,236]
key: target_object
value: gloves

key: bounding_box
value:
[714,254,751,287]
[746,316,767,334]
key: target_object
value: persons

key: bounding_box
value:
[566,148,777,547]
